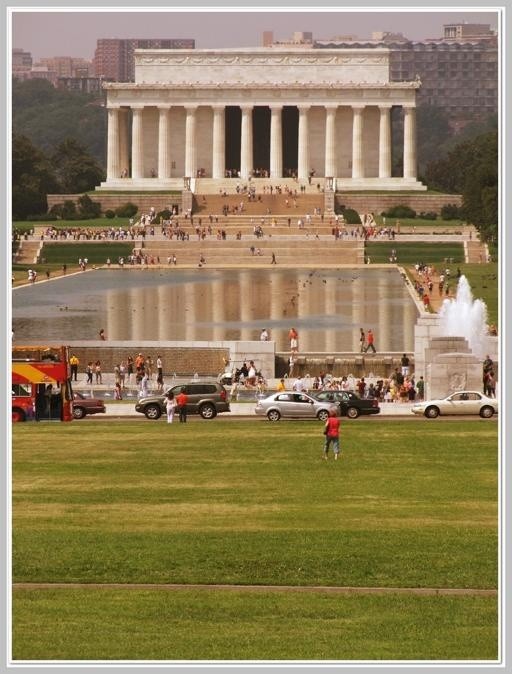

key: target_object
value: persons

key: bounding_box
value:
[324,409,341,460]
[412,260,461,305]
[121,167,316,184]
[11,326,497,423]
[12,183,397,289]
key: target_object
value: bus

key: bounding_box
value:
[12,343,74,424]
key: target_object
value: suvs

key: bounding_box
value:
[132,380,233,420]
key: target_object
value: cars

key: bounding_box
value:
[72,391,107,420]
[313,390,382,420]
[217,371,267,390]
[253,391,341,422]
[409,389,498,420]
